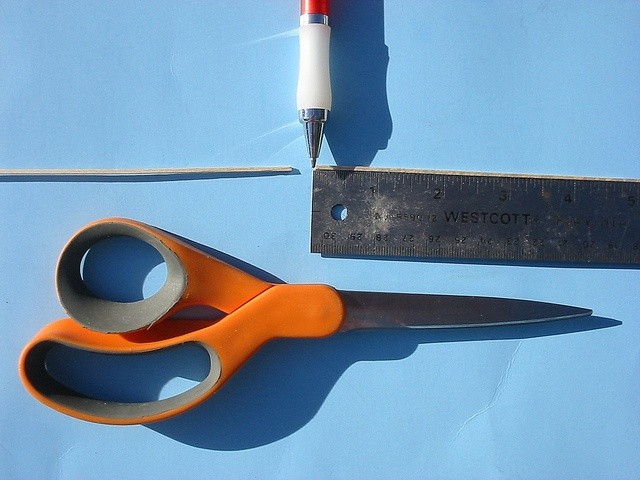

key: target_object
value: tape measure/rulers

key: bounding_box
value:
[310,166,640,270]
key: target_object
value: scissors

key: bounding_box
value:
[17,219,592,426]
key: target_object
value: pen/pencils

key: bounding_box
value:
[298,0,332,168]
